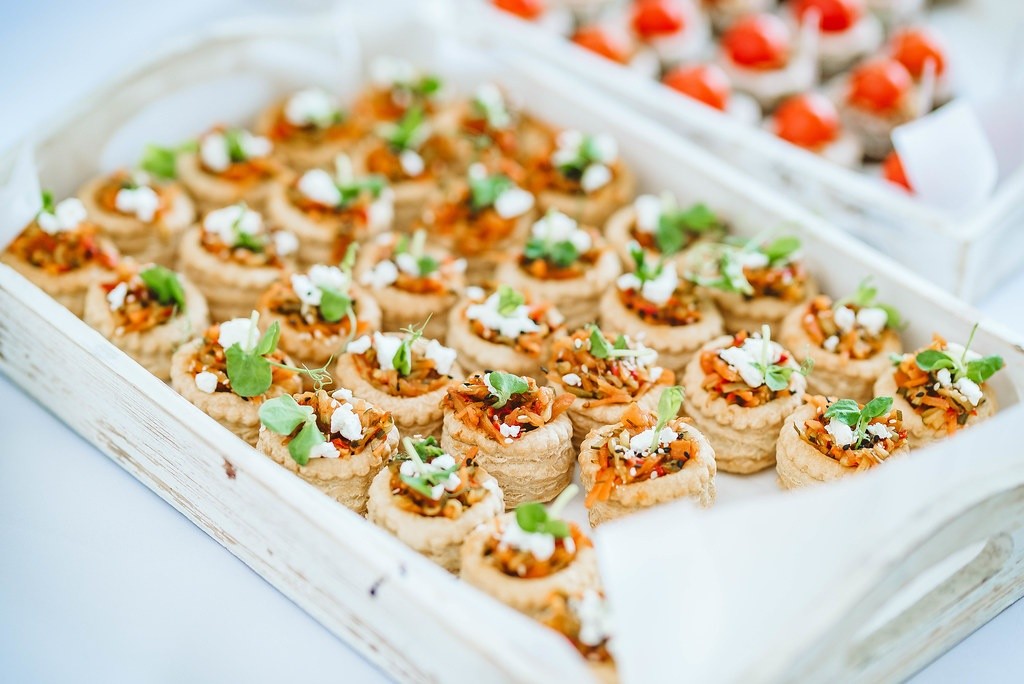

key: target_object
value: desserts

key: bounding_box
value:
[0,1,996,664]
